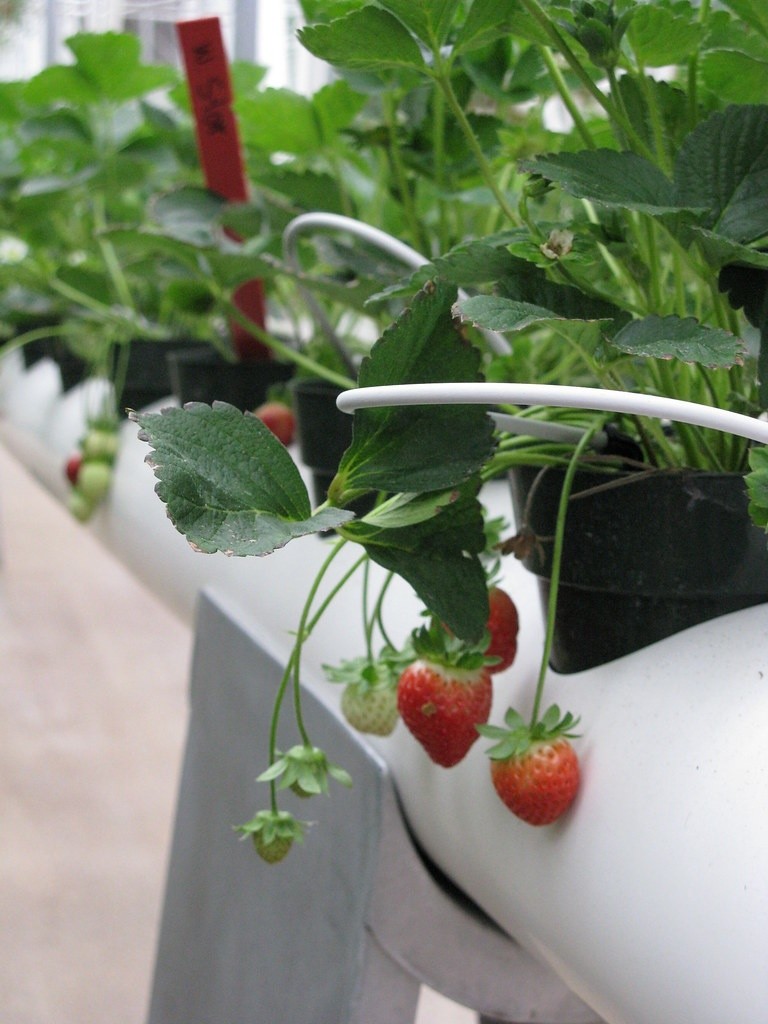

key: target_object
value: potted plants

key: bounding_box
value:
[0,0,768,865]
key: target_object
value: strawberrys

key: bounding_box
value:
[60,398,579,865]
